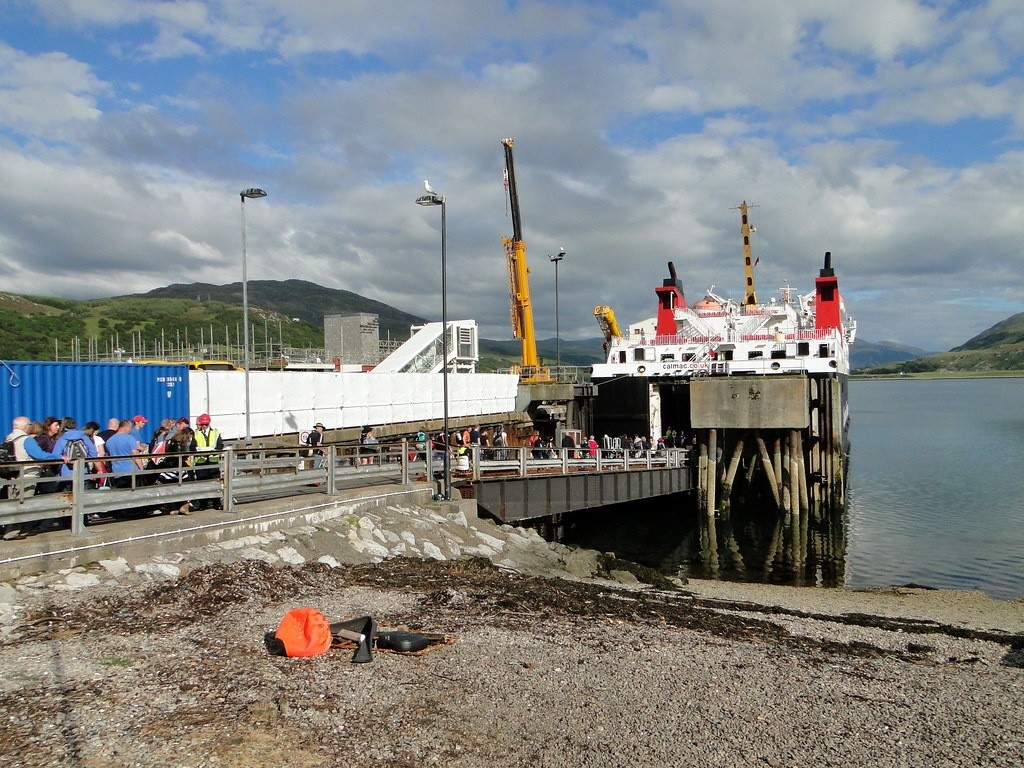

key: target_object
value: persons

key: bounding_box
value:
[415,423,702,460]
[0,413,224,541]
[306,423,328,487]
[359,426,380,465]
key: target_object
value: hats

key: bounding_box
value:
[362,426,373,433]
[132,416,148,423]
[312,423,325,431]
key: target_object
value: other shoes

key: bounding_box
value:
[169,508,182,515]
[193,505,207,510]
[213,502,222,509]
[179,505,192,515]
[4,534,25,540]
[18,530,37,536]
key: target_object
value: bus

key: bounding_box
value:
[134,360,243,371]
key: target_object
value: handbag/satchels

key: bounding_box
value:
[534,437,543,447]
[582,441,589,454]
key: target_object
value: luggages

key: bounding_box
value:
[307,450,328,487]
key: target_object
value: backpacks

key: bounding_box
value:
[62,436,90,470]
[0,435,27,480]
[493,432,503,447]
[456,429,469,445]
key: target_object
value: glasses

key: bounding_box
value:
[200,423,209,426]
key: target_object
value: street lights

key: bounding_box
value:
[546,248,566,381]
[414,194,478,522]
[239,188,268,459]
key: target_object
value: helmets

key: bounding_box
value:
[199,414,211,424]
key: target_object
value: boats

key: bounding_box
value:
[589,198,858,457]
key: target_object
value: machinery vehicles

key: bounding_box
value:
[500,137,558,384]
